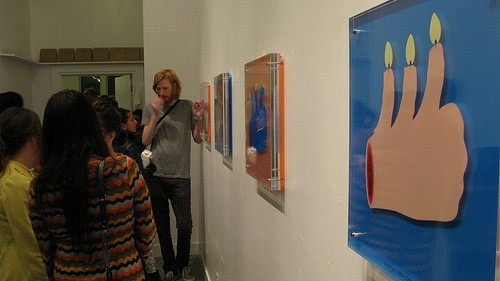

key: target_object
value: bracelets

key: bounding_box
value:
[194,117,202,121]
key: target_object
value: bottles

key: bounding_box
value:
[141,150,152,169]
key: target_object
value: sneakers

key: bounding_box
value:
[180,267,195,281]
[162,271,178,281]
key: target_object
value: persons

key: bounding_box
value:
[27,89,158,281]
[0,91,23,107]
[0,107,48,281]
[141,68,205,281]
[84,86,156,183]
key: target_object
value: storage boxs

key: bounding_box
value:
[110,48,125,61]
[75,49,93,62]
[125,47,140,61]
[57,49,75,62]
[93,48,109,62]
[39,49,57,62]
